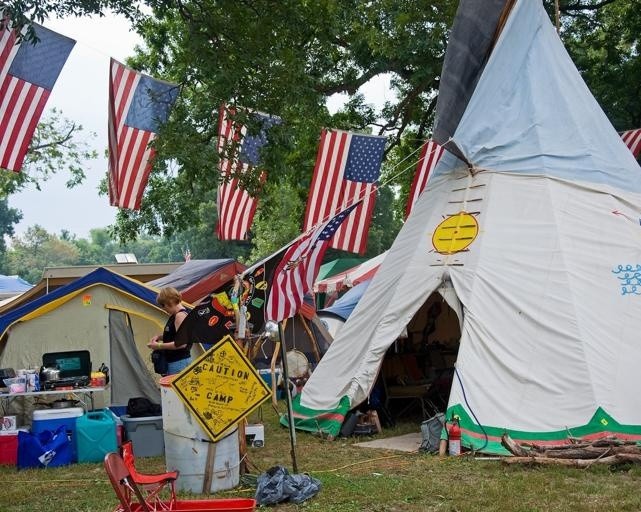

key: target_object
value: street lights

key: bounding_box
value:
[418,413,444,453]
[18,425,73,467]
[152,350,168,374]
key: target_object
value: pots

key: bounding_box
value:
[32,398,80,409]
[42,366,61,381]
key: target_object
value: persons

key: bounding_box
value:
[147,287,195,375]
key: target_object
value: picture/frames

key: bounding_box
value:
[0,382,114,426]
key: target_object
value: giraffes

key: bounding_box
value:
[44,375,91,388]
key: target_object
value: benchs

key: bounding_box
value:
[107,55,180,211]
[619,130,641,160]
[265,201,363,323]
[181,240,192,262]
[406,141,448,221]
[171,245,297,341]
[1,4,77,174]
[215,103,282,241]
[303,127,387,256]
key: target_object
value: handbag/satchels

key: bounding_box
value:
[157,343,162,350]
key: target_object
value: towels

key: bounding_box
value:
[158,372,242,494]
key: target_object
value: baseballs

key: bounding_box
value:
[444,411,461,457]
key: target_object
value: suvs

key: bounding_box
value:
[76,413,118,465]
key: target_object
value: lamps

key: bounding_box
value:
[119,439,180,510]
[381,339,461,421]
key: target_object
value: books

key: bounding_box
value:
[121,413,164,458]
[31,406,85,471]
[257,366,284,402]
[1,425,30,465]
